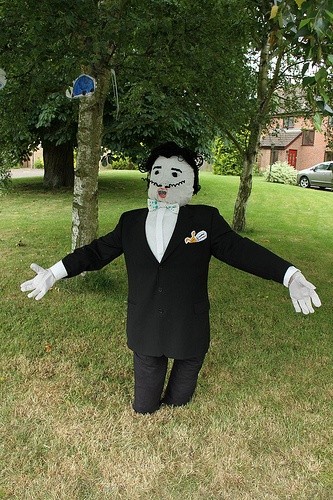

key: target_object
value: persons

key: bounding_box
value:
[20,139,321,414]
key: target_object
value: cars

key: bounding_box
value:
[297,162,333,191]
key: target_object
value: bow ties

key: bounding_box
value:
[147,199,179,216]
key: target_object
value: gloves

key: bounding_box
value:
[289,273,322,315]
[18,262,55,301]
